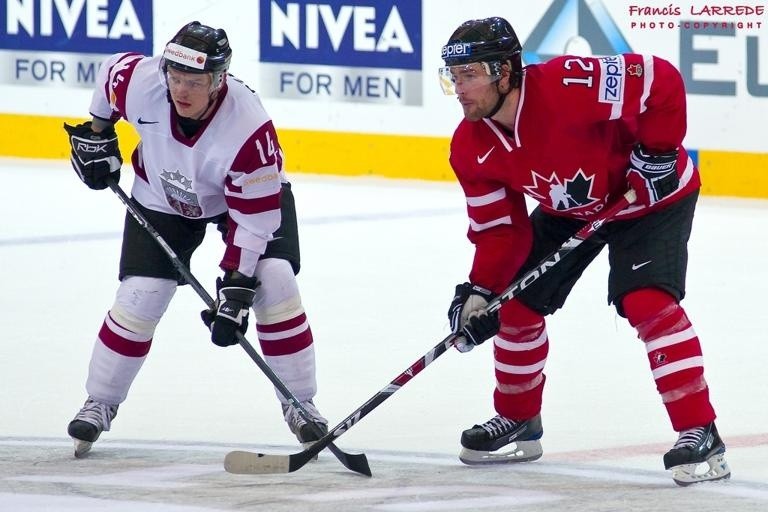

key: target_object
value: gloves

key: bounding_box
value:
[447,282,501,353]
[62,122,124,191]
[625,144,684,210]
[201,275,263,351]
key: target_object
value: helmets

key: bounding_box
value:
[161,21,233,77]
[441,16,523,69]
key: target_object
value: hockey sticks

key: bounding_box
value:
[105,175,374,478]
[224,189,640,473]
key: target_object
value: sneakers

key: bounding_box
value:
[663,421,727,470]
[460,412,545,449]
[68,396,119,443]
[280,398,329,444]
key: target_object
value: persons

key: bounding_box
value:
[440,18,727,470]
[63,19,331,443]
